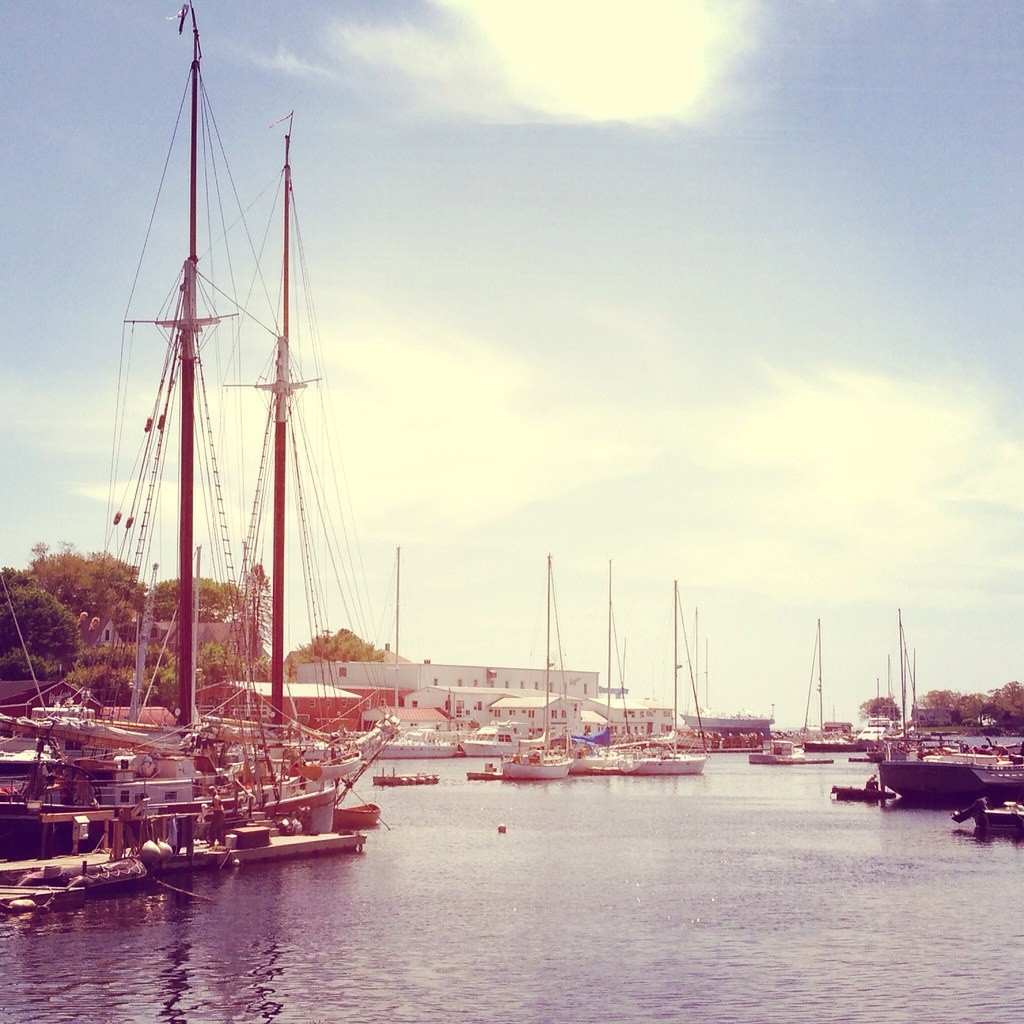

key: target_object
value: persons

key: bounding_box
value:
[197,784,227,847]
[866,773,876,787]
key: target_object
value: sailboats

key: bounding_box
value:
[1,2,1024,914]
